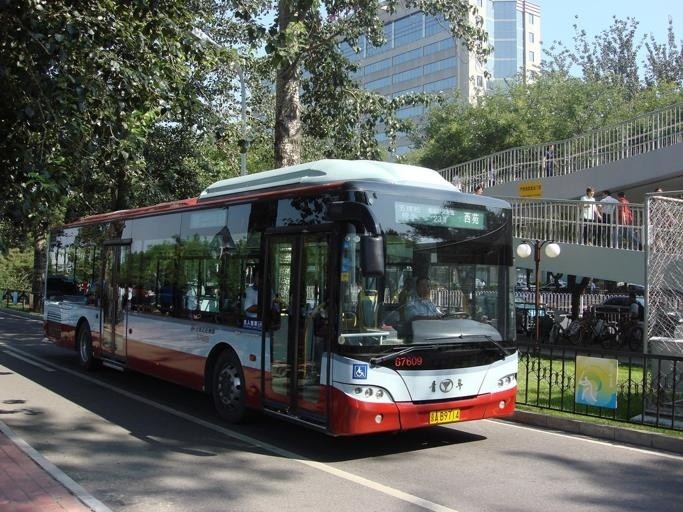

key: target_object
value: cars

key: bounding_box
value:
[587,296,641,319]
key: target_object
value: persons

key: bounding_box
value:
[577,187,602,246]
[616,192,641,250]
[628,293,640,320]
[472,185,483,195]
[244,267,261,318]
[487,160,497,186]
[597,190,619,247]
[82,279,159,312]
[405,277,443,324]
[543,144,559,177]
[452,169,462,192]
[591,280,596,294]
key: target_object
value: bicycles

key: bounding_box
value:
[532,315,643,351]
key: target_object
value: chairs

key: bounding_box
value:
[358,288,379,333]
[400,277,417,317]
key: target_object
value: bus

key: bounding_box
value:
[39,159,519,442]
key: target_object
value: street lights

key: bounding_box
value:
[515,235,561,360]
